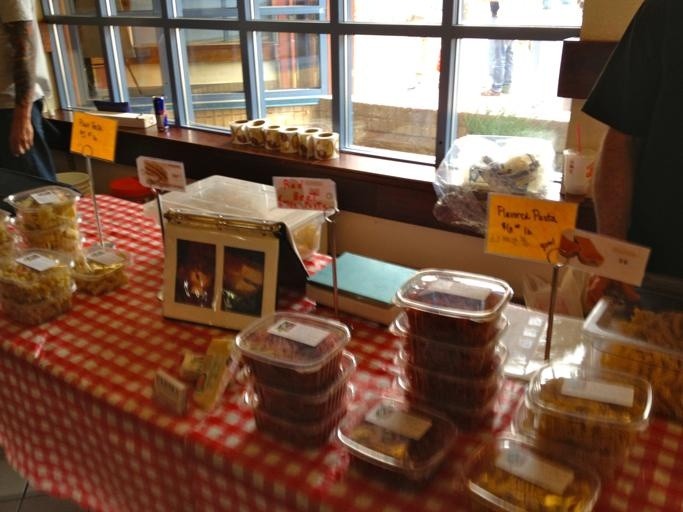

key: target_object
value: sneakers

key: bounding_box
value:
[481,90,500,96]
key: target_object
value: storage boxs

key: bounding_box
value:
[143,174,334,262]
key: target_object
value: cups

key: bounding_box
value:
[562,148,595,195]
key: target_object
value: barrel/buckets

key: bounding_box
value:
[56,172,92,196]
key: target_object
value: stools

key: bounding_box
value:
[56,171,93,193]
[108,176,152,203]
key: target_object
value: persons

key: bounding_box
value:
[480,10,515,96]
[580,0,683,316]
[0,0,56,181]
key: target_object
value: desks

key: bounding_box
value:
[0,193,683,512]
